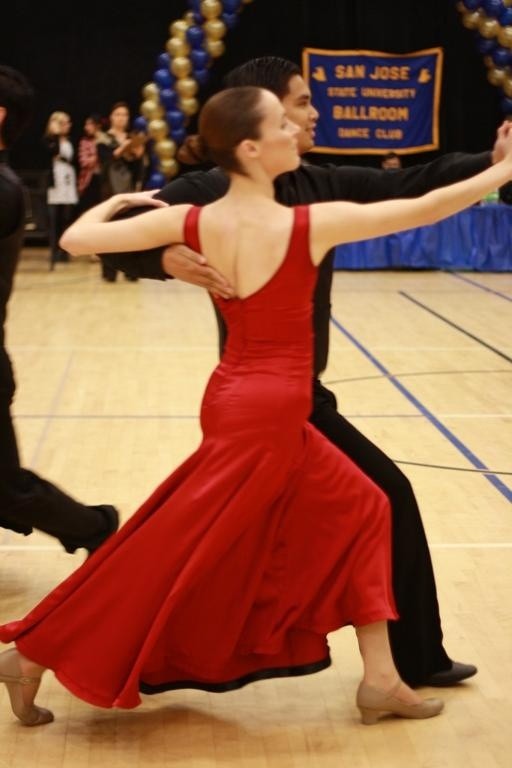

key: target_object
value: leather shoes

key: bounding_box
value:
[400,660,478,689]
[88,505,117,549]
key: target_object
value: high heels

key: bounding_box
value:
[358,680,445,724]
[0,649,54,726]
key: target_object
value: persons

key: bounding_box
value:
[0,81,118,564]
[0,81,512,728]
[33,100,155,287]
[381,150,401,172]
[98,57,512,689]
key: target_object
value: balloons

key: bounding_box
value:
[136,1,242,189]
[461,0,512,99]
